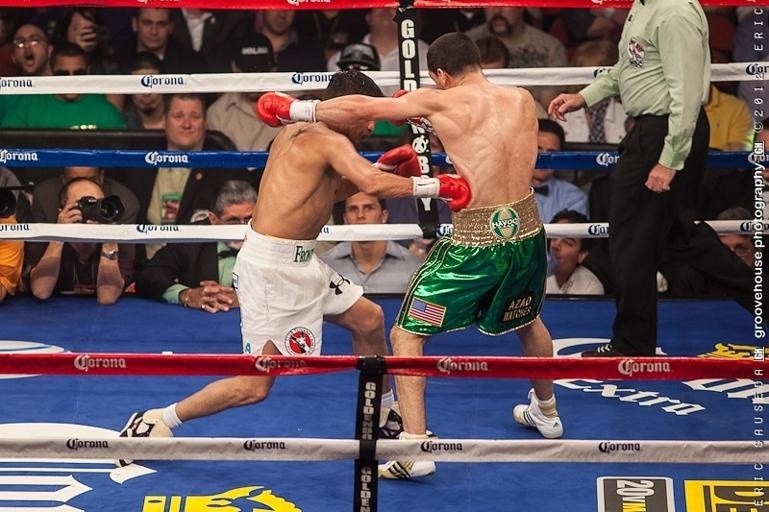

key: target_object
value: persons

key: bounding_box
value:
[115,67,439,469]
[545,1,767,362]
[259,30,564,481]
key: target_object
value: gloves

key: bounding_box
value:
[409,172,474,213]
[370,143,422,177]
[256,90,321,127]
[387,89,434,137]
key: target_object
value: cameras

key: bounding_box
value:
[77,196,124,223]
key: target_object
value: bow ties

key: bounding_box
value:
[218,248,241,259]
[532,183,550,197]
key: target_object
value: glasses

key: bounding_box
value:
[12,34,49,48]
[53,68,89,76]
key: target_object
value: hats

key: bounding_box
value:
[335,42,382,71]
[232,31,279,73]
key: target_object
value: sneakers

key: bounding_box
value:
[581,342,626,358]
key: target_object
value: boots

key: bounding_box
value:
[113,407,176,469]
[377,432,439,480]
[378,400,441,440]
[512,387,564,439]
